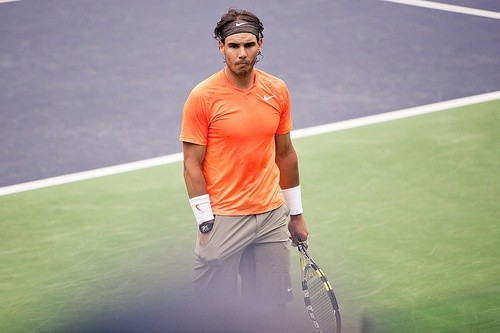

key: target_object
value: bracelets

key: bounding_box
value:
[281,183,303,216]
[188,194,215,225]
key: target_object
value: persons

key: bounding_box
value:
[177,10,309,314]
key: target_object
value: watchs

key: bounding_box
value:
[198,221,216,233]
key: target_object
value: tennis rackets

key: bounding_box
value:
[298,244,341,333]
